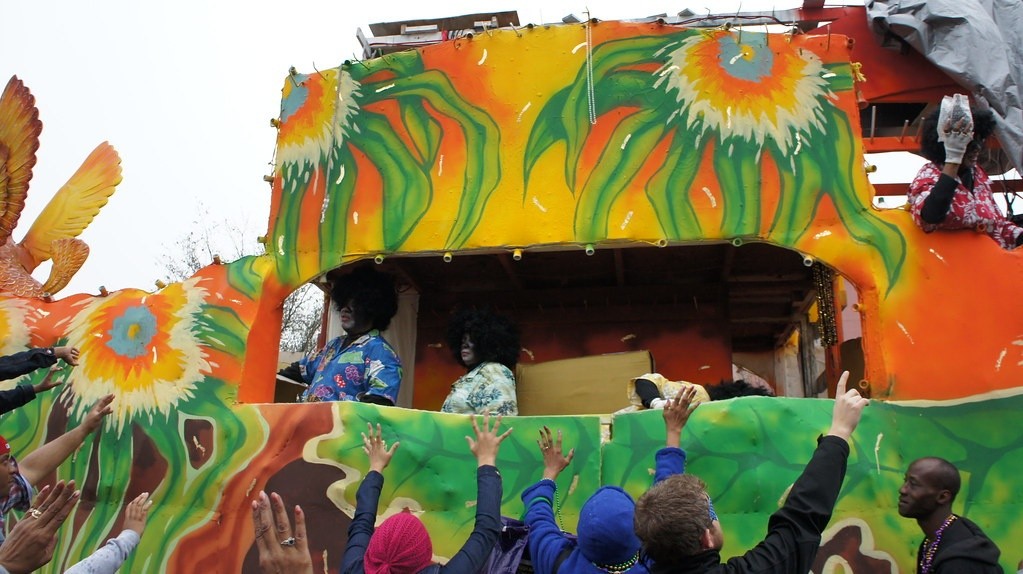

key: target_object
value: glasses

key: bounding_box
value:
[971,139,987,147]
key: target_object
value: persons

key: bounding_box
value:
[277,266,402,406]
[907,103,1023,249]
[898,457,1004,574]
[440,306,522,416]
[0,479,80,574]
[339,408,513,574]
[633,370,870,574]
[521,385,701,574]
[252,491,313,574]
[0,346,80,415]
[63,491,153,574]
[0,394,116,546]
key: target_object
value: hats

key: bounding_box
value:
[922,91,995,158]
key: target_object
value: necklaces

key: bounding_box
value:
[537,478,639,574]
[920,515,957,574]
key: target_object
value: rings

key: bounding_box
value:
[281,537,295,546]
[32,509,42,519]
[29,508,34,512]
[545,447,548,449]
